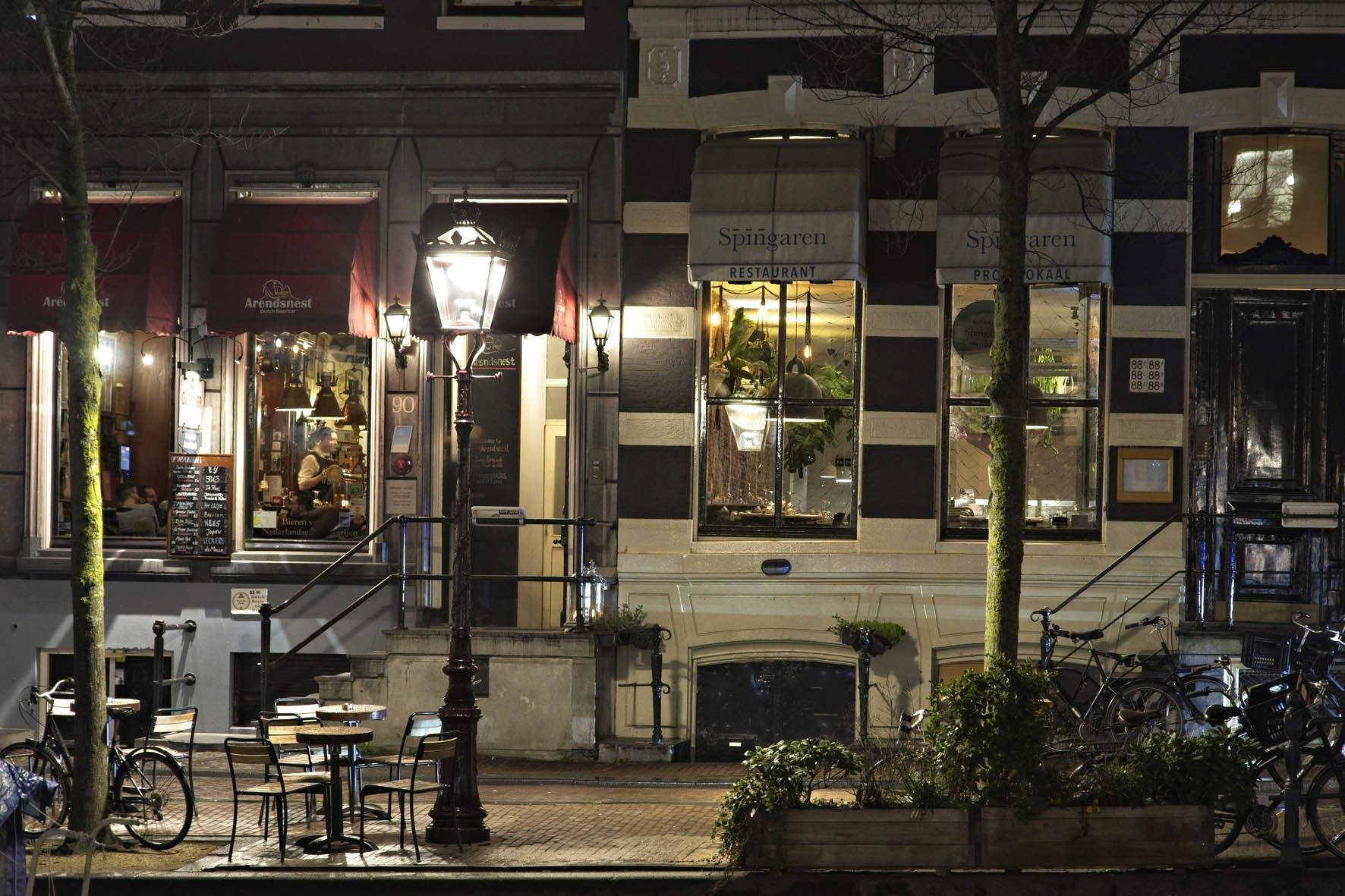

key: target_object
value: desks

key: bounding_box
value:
[68,698,140,814]
[292,725,379,855]
[314,704,393,819]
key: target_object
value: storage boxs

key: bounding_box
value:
[1243,670,1314,746]
[1242,629,1294,672]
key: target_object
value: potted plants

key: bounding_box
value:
[591,605,661,651]
[707,614,1265,891]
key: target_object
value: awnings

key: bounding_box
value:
[687,137,866,289]
[936,132,1112,286]
[207,195,379,337]
[6,199,183,334]
[409,202,580,343]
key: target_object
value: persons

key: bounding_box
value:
[298,426,338,502]
[116,482,168,535]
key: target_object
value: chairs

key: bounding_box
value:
[45,692,199,818]
[223,698,465,863]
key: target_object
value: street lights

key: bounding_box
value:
[413,187,519,840]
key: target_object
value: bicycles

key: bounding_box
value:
[855,711,945,806]
[1036,608,1345,859]
[0,678,196,850]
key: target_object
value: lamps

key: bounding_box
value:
[578,292,613,370]
[307,336,346,421]
[339,338,371,423]
[769,281,826,424]
[1026,382,1049,429]
[275,335,314,410]
[385,294,418,371]
